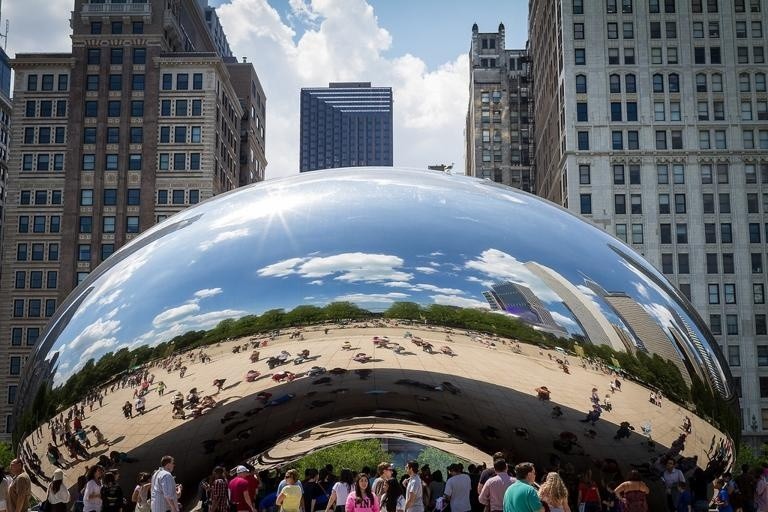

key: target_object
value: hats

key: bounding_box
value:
[237,466,249,473]
[53,469,63,481]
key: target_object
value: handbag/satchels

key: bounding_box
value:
[435,497,449,511]
[578,503,585,512]
[422,481,430,507]
[39,500,50,512]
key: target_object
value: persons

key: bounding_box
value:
[0,451,768,512]
[534,350,736,491]
[122,348,228,420]
[234,329,370,412]
[21,389,106,487]
[372,331,461,399]
